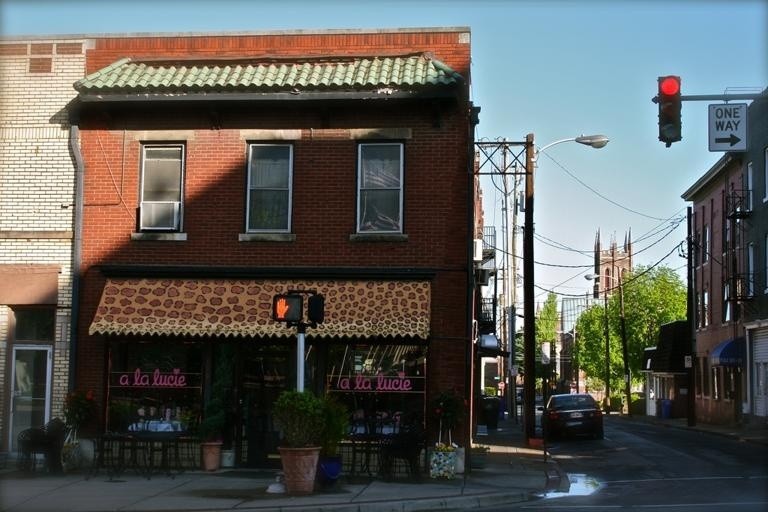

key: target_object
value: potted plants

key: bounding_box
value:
[276,387,326,497]
[199,358,232,471]
[318,396,347,488]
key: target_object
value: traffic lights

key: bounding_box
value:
[274,295,303,322]
[656,75,682,143]
[307,293,324,324]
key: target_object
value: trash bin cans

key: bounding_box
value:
[484,396,501,429]
[656,399,672,417]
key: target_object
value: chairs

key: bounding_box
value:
[15,418,199,486]
[351,431,422,480]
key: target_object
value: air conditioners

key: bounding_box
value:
[473,237,484,262]
[475,266,491,286]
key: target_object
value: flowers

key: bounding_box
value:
[434,443,455,452]
[428,391,470,444]
[62,386,99,415]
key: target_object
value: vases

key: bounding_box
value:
[429,451,457,480]
[218,448,237,468]
[426,444,466,474]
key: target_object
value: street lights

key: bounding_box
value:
[584,266,636,418]
[556,324,577,380]
[520,131,613,444]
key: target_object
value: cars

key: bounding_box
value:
[537,394,605,444]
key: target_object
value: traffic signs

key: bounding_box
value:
[707,103,748,153]
